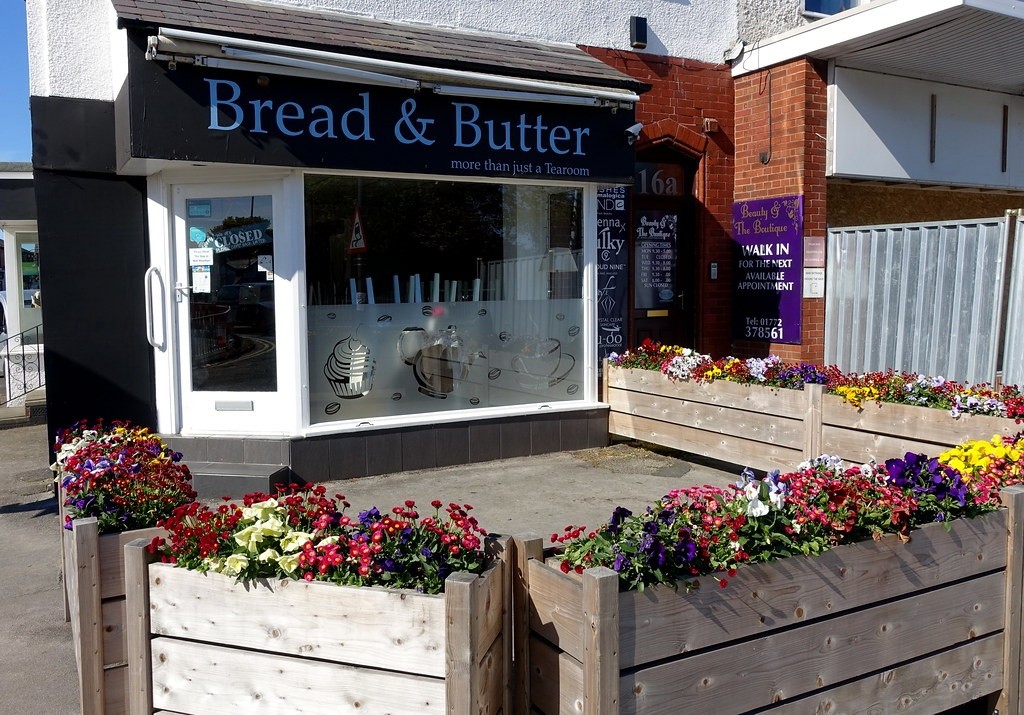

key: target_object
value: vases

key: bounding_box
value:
[604,358,1024,474]
[124,534,514,715]
[513,485,1024,715]
[58,464,168,715]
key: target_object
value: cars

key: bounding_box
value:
[0,290,44,376]
[216,283,274,332]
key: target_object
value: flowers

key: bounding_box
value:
[45,417,199,535]
[608,338,1024,425]
[145,483,486,592]
[549,428,1024,590]
